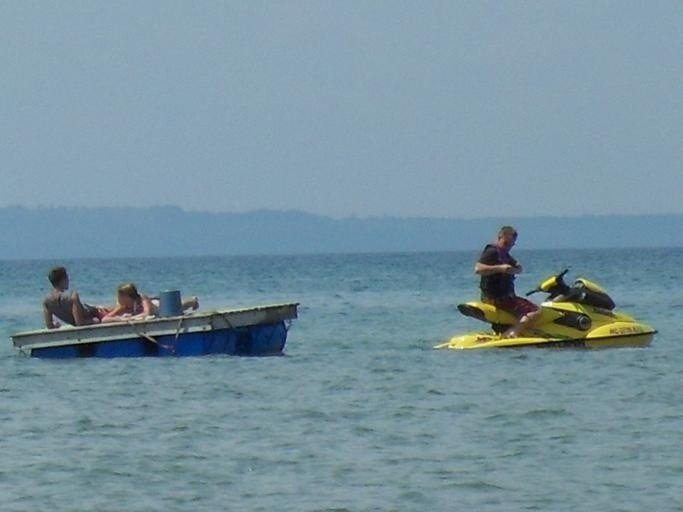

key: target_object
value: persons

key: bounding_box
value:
[40,267,113,330]
[100,282,200,322]
[473,224,542,338]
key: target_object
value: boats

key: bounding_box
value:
[8,300,300,360]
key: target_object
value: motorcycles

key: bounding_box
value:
[433,267,658,352]
